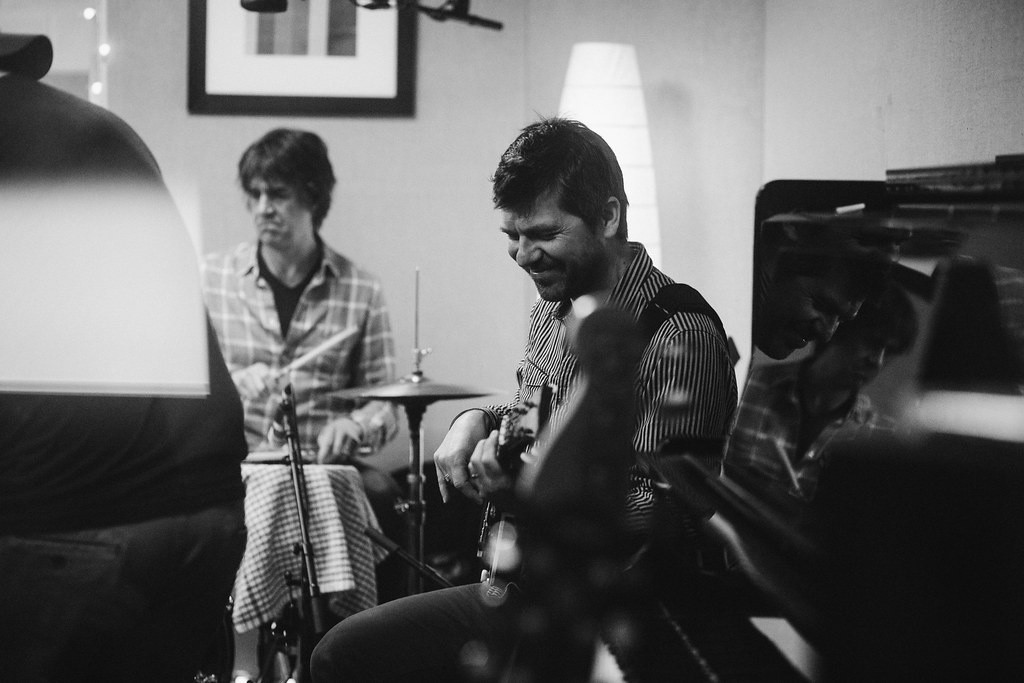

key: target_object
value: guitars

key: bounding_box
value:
[477,289,630,615]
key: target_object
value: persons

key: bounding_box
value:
[729,223,920,492]
[309,113,737,682]
[0,28,248,683]
[196,126,400,683]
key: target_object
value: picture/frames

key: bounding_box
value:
[185,0,419,121]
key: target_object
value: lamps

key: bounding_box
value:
[0,32,211,398]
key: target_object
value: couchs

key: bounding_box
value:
[0,329,407,683]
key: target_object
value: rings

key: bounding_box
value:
[443,474,450,482]
[471,473,478,479]
[344,445,352,451]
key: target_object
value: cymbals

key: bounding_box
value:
[322,371,502,403]
